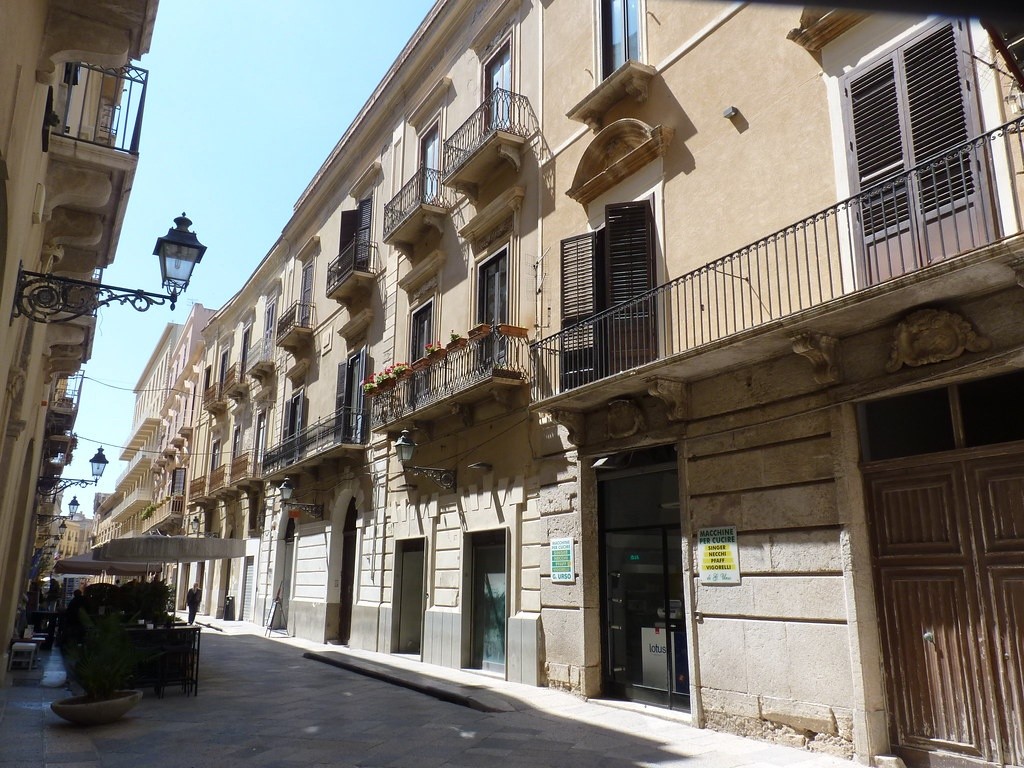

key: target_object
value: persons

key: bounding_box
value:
[42,578,60,612]
[186,582,202,626]
[66,590,84,606]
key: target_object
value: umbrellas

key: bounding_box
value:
[91,537,245,623]
[52,538,164,585]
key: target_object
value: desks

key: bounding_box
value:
[29,609,203,699]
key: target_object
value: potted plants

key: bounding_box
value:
[373,367,396,391]
[425,341,446,364]
[390,362,412,381]
[467,323,491,339]
[495,323,528,337]
[361,372,379,397]
[445,329,467,351]
[412,357,429,370]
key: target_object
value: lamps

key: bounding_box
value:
[35,444,110,495]
[191,515,219,538]
[8,212,207,330]
[277,474,326,522]
[35,495,81,527]
[394,427,458,495]
[34,543,57,555]
[38,520,67,540]
